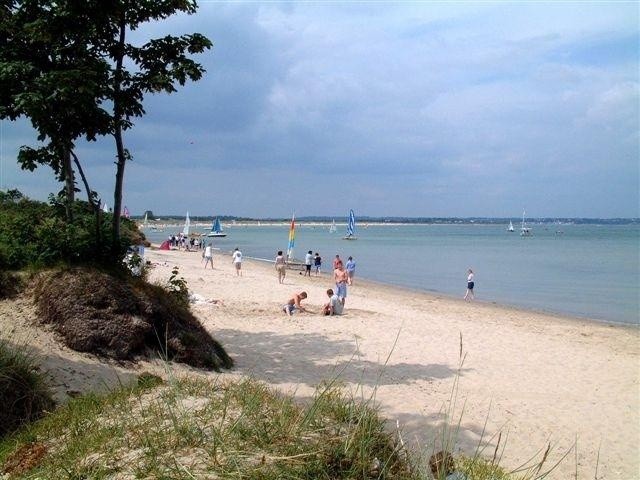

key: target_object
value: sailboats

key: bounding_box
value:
[554,217,564,234]
[507,219,513,231]
[208,218,226,237]
[330,219,336,233]
[284,209,306,266]
[343,209,357,240]
[170,212,206,246]
[520,208,533,236]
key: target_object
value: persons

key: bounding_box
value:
[430,451,466,480]
[232,248,242,276]
[304,251,355,317]
[464,269,474,300]
[133,235,213,269]
[283,291,307,316]
[274,251,286,284]
